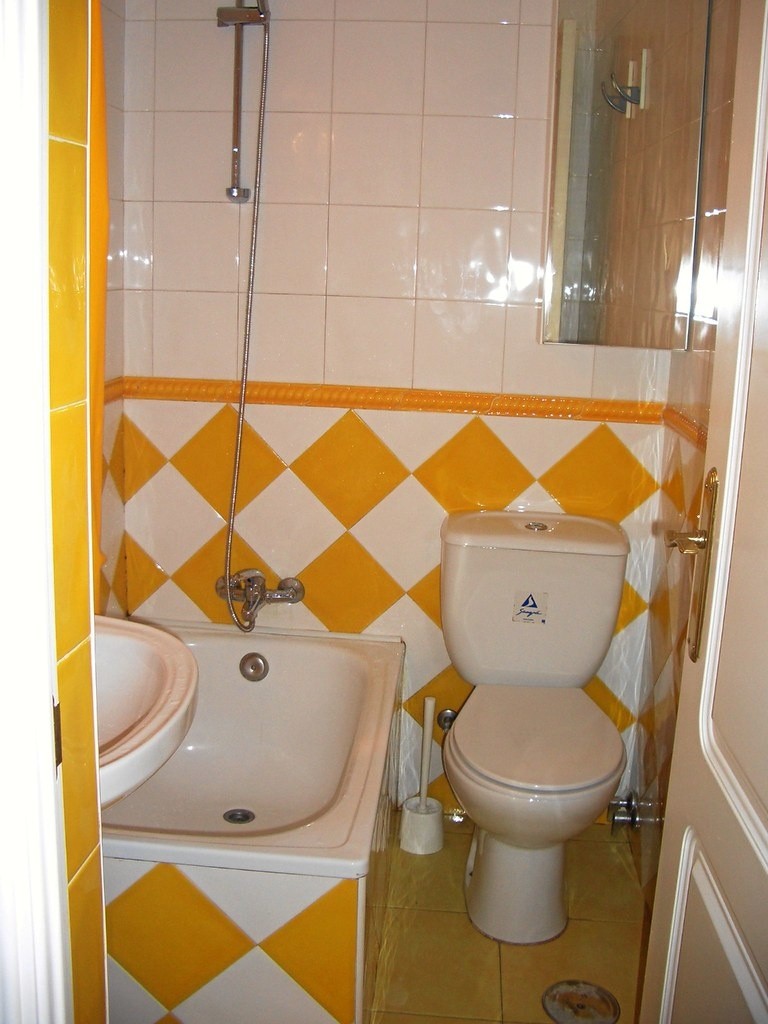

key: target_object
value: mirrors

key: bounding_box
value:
[536,0,709,351]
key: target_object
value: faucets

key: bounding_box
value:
[214,568,307,619]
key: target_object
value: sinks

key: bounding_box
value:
[96,617,198,807]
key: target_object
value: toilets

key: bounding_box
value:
[439,511,630,943]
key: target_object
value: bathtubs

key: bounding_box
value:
[98,620,407,1023]
